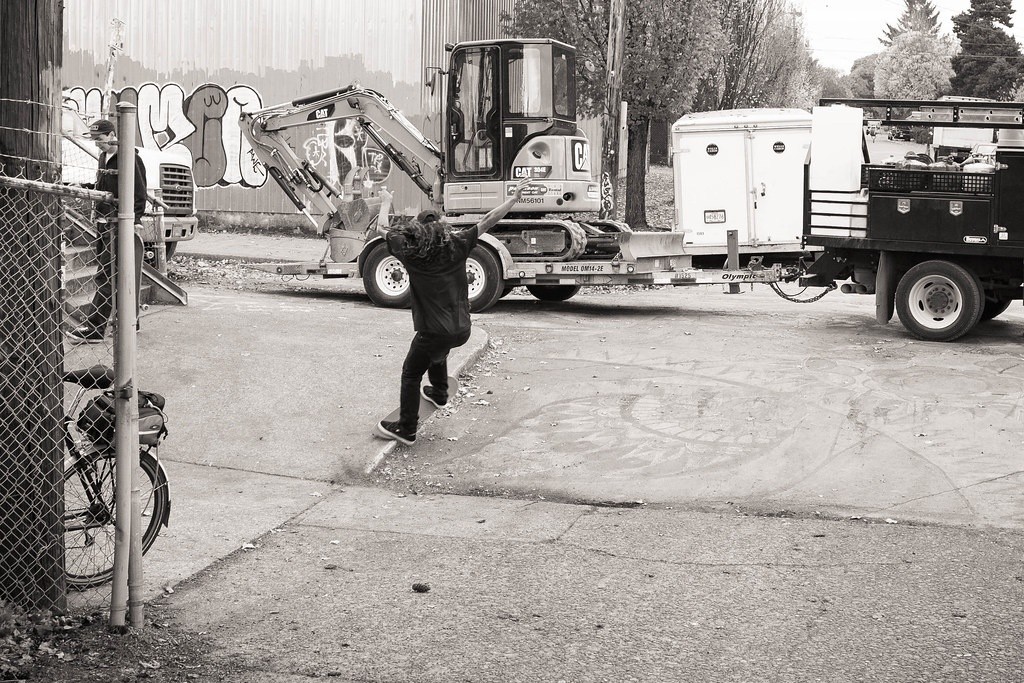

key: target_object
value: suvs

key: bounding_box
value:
[60,105,200,263]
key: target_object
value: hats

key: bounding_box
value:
[83,120,115,135]
[419,209,440,227]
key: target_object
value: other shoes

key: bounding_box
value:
[65,327,107,345]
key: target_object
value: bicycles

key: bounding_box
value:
[63,360,172,590]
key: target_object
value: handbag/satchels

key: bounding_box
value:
[79,388,166,447]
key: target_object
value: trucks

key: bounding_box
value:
[797,98,1024,343]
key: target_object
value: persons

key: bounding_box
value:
[376,174,532,446]
[66,119,147,342]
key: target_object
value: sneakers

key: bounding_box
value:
[378,419,417,446]
[421,384,447,409]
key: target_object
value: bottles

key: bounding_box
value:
[67,421,92,455]
[887,155,896,165]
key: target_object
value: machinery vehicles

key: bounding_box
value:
[237,37,801,339]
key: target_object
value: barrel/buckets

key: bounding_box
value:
[946,164,959,190]
[928,162,946,190]
[895,162,928,189]
[962,166,993,192]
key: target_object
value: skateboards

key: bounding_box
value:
[370,375,460,440]
